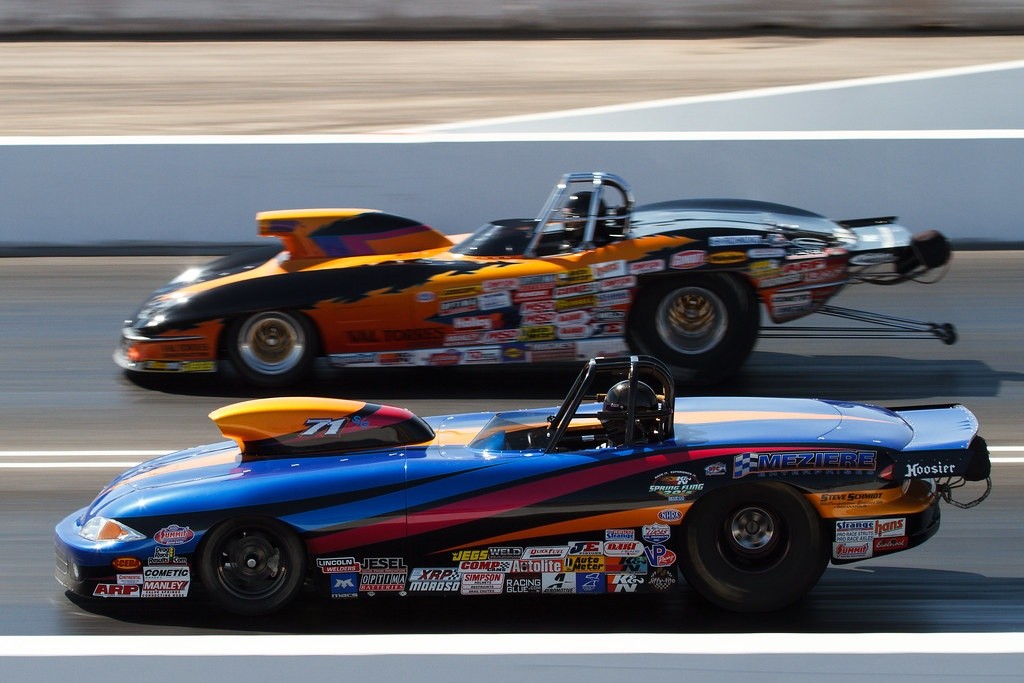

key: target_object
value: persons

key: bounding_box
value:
[561,191,607,253]
[597,380,658,449]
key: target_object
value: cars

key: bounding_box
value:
[50,353,995,627]
[112,172,960,398]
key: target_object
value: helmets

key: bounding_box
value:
[560,192,607,237]
[601,380,658,441]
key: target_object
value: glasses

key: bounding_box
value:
[561,215,580,229]
